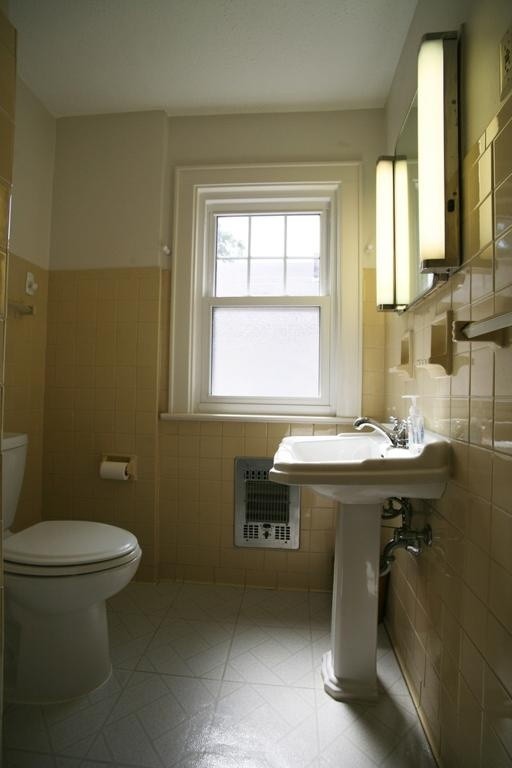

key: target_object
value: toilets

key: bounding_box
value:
[0,429,143,705]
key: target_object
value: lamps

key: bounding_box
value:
[416,27,463,280]
[395,154,411,317]
[374,158,396,313]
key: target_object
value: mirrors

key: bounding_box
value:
[394,86,437,322]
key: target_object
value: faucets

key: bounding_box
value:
[352,416,407,448]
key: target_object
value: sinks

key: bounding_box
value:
[268,434,460,506]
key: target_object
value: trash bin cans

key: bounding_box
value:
[329,550,391,625]
